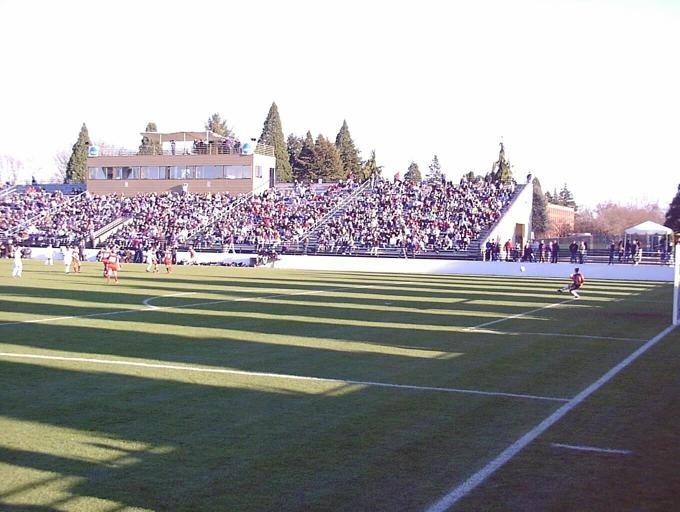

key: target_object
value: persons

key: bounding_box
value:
[169,139,241,155]
[0,169,518,284]
[557,268,585,300]
[519,236,673,264]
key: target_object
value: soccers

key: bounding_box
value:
[519,266,524,273]
